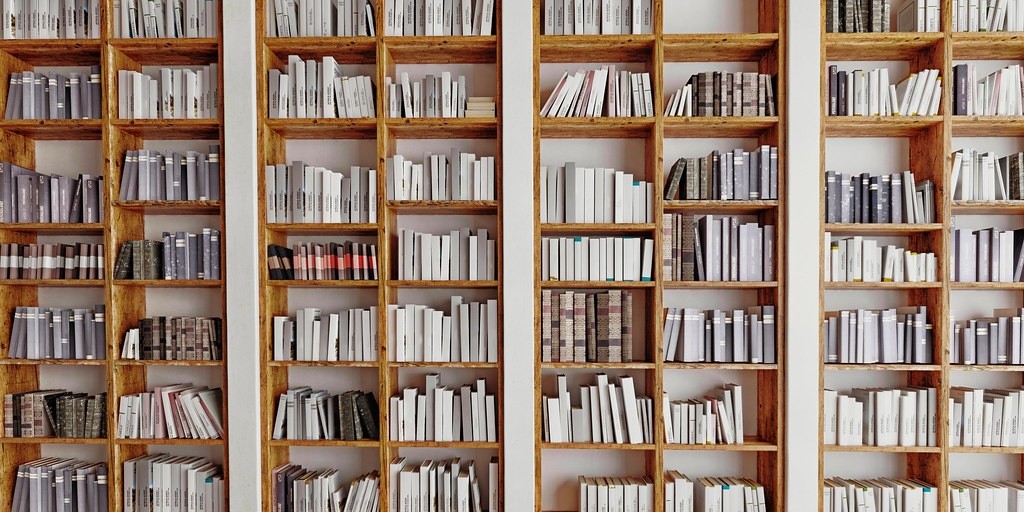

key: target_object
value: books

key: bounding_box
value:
[0,1,1023,512]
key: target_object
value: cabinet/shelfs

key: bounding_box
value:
[534,0,788,512]
[257,1,502,512]
[0,0,225,512]
[820,0,1024,512]
